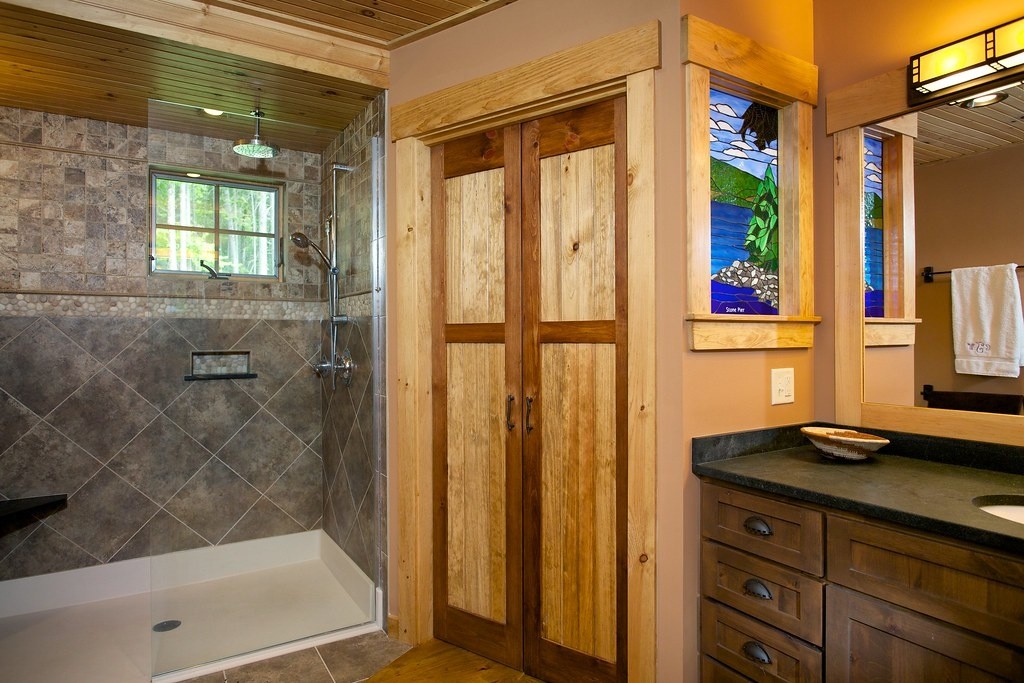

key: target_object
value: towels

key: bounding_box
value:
[951,263,1024,379]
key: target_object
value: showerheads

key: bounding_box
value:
[233,139,280,159]
[290,232,331,269]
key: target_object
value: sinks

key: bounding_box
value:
[971,494,1024,525]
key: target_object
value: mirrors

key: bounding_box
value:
[859,78,1024,418]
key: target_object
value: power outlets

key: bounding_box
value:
[772,368,795,405]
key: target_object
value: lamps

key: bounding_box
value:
[905,16,1024,108]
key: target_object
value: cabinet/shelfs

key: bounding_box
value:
[697,478,1024,683]
